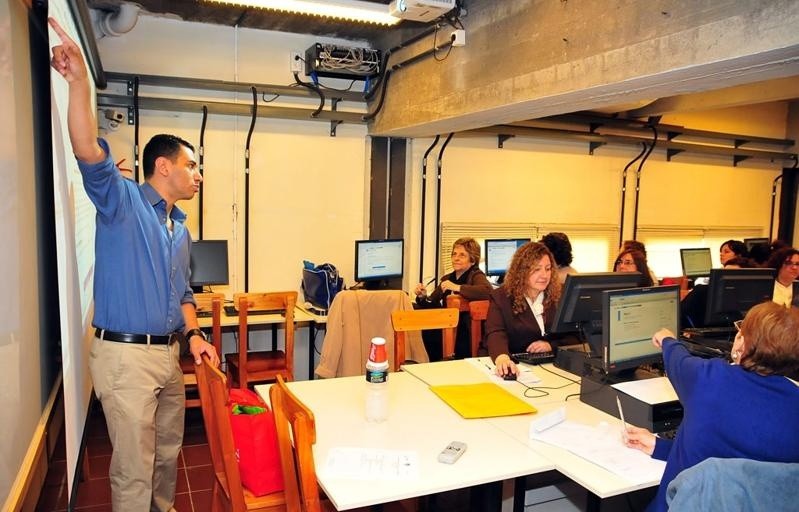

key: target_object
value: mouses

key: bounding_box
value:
[281,312,295,318]
[503,367,517,380]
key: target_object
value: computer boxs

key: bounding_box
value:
[580,373,683,434]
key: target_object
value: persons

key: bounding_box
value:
[745,237,788,267]
[414,236,493,365]
[678,257,759,331]
[621,240,661,287]
[43,15,223,512]
[758,247,798,307]
[719,240,749,266]
[540,231,580,283]
[611,247,694,329]
[485,241,566,379]
[621,300,798,512]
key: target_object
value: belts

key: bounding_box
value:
[94,327,179,344]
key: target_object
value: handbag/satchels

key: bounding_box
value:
[300,263,343,311]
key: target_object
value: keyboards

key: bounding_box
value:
[512,352,555,365]
[223,306,295,316]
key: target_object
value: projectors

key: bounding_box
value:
[389,0,456,25]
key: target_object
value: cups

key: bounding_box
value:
[367,336,390,367]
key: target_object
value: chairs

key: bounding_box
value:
[194,353,286,512]
[469,300,489,357]
[225,291,298,388]
[179,293,226,408]
[269,373,321,511]
[391,308,459,371]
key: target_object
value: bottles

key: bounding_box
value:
[366,365,389,423]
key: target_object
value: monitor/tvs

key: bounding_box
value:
[550,237,799,385]
[190,240,229,293]
[485,239,531,286]
[350,239,404,290]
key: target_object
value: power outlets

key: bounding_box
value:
[451,30,465,46]
[290,52,303,72]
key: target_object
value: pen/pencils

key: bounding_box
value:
[616,396,628,442]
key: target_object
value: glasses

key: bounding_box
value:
[784,260,799,266]
[416,278,445,308]
[618,260,634,267]
[734,320,745,343]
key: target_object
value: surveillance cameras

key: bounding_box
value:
[104,109,124,123]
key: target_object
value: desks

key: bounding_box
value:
[254,357,667,511]
[296,300,328,380]
[180,301,316,381]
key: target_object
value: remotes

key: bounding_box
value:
[438,441,466,464]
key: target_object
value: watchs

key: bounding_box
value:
[185,328,208,345]
